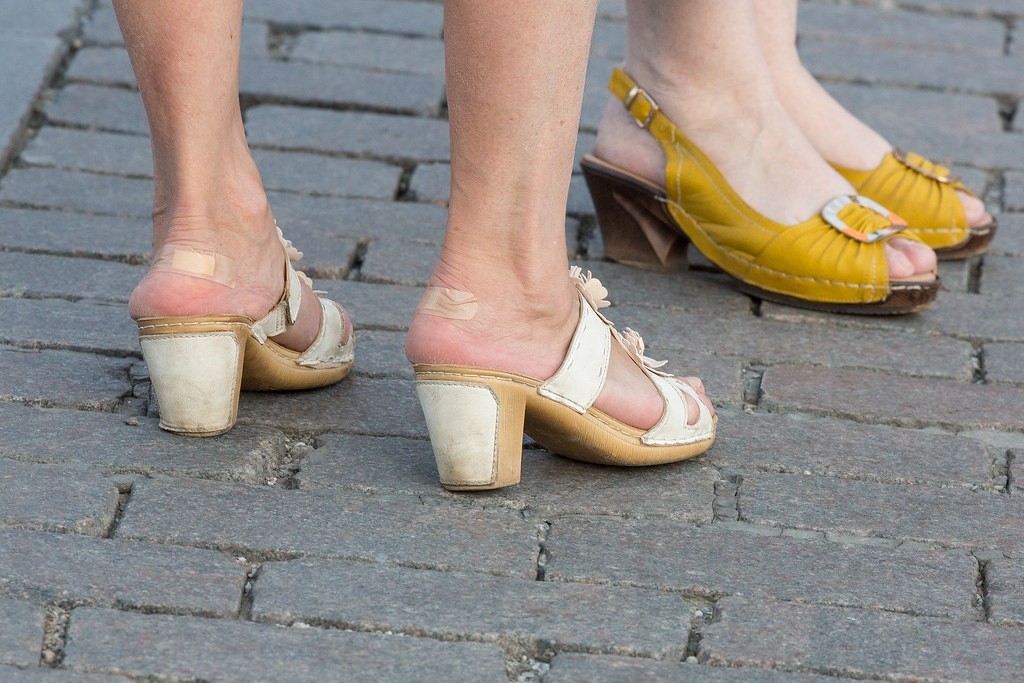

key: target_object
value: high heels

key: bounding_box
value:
[412,264,719,490]
[580,68,941,315]
[822,145,999,263]
[138,218,355,437]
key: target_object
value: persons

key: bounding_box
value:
[579,0,1001,316]
[111,0,718,495]
